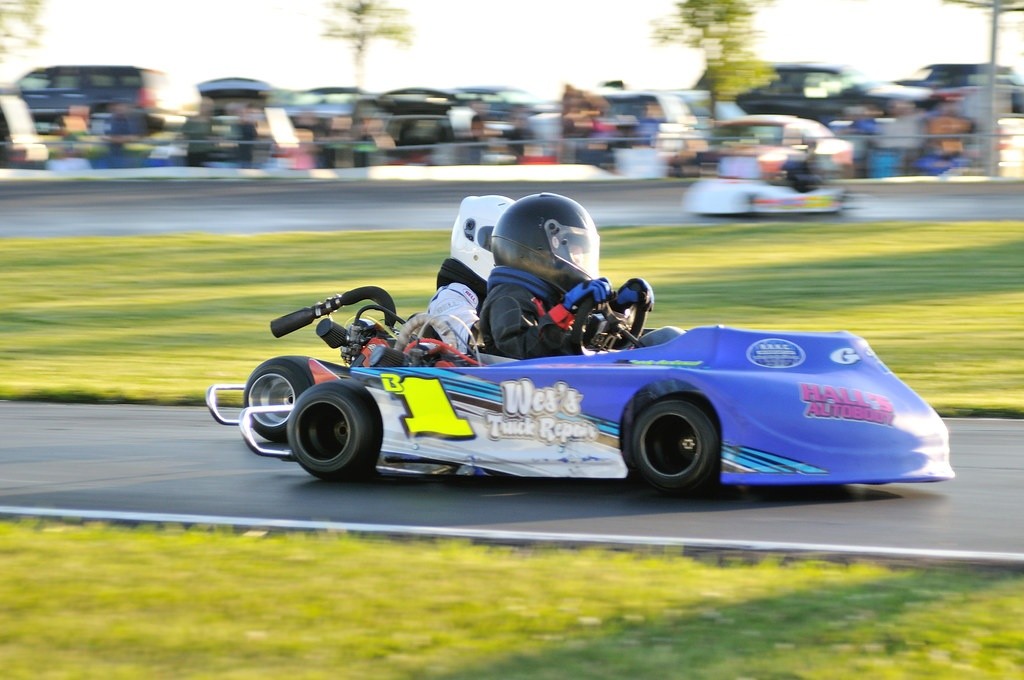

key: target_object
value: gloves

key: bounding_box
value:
[551,277,655,328]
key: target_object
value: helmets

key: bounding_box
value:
[451,194,518,280]
[492,193,601,289]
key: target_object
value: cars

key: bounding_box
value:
[0,62,1023,174]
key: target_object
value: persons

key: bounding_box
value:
[480,192,655,359]
[426,194,516,355]
